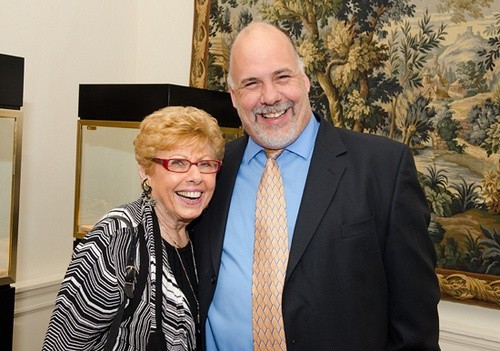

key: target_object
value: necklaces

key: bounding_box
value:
[158,218,202,334]
[161,225,189,248]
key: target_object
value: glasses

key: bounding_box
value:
[146,158,223,174]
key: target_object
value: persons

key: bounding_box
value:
[185,23,443,351]
[41,107,224,351]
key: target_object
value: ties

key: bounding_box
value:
[252,148,289,350]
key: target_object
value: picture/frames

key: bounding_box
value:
[189,0,500,311]
